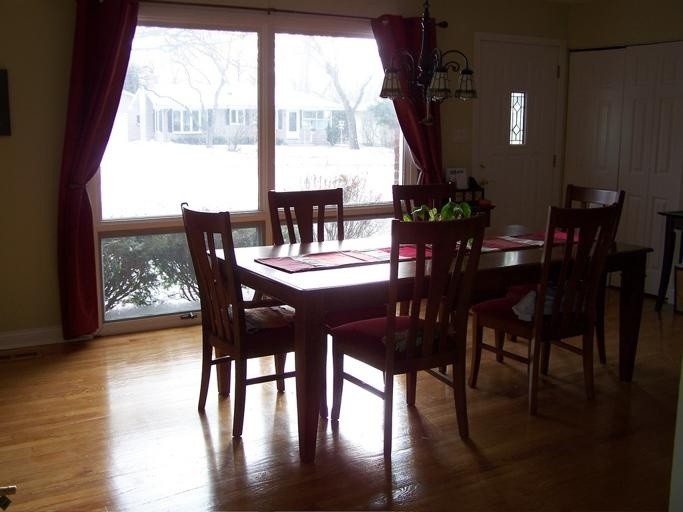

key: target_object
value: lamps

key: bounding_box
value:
[379,0,480,126]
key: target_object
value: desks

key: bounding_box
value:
[206,223,654,463]
